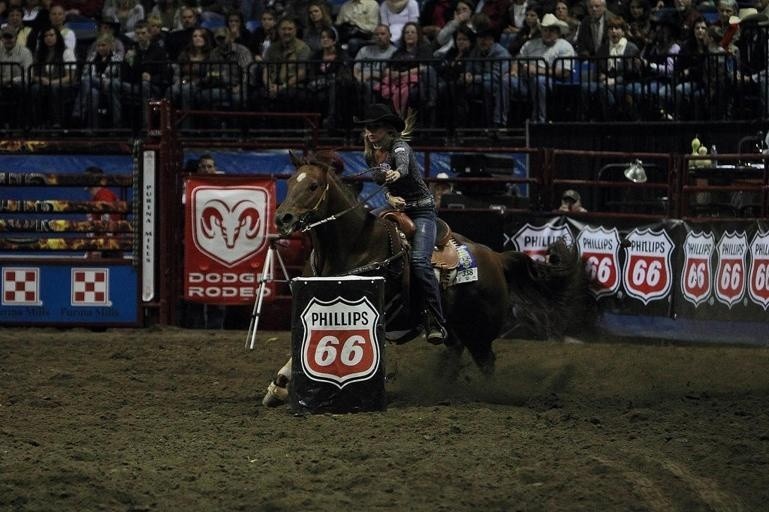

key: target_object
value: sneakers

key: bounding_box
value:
[425,321,446,341]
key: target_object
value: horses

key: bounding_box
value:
[263,151,605,407]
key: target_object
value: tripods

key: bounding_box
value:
[243,250,293,353]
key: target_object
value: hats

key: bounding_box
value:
[350,102,407,135]
[214,26,230,38]
[535,12,570,37]
[561,188,582,203]
[728,7,769,26]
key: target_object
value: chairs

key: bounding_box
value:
[63,11,607,124]
